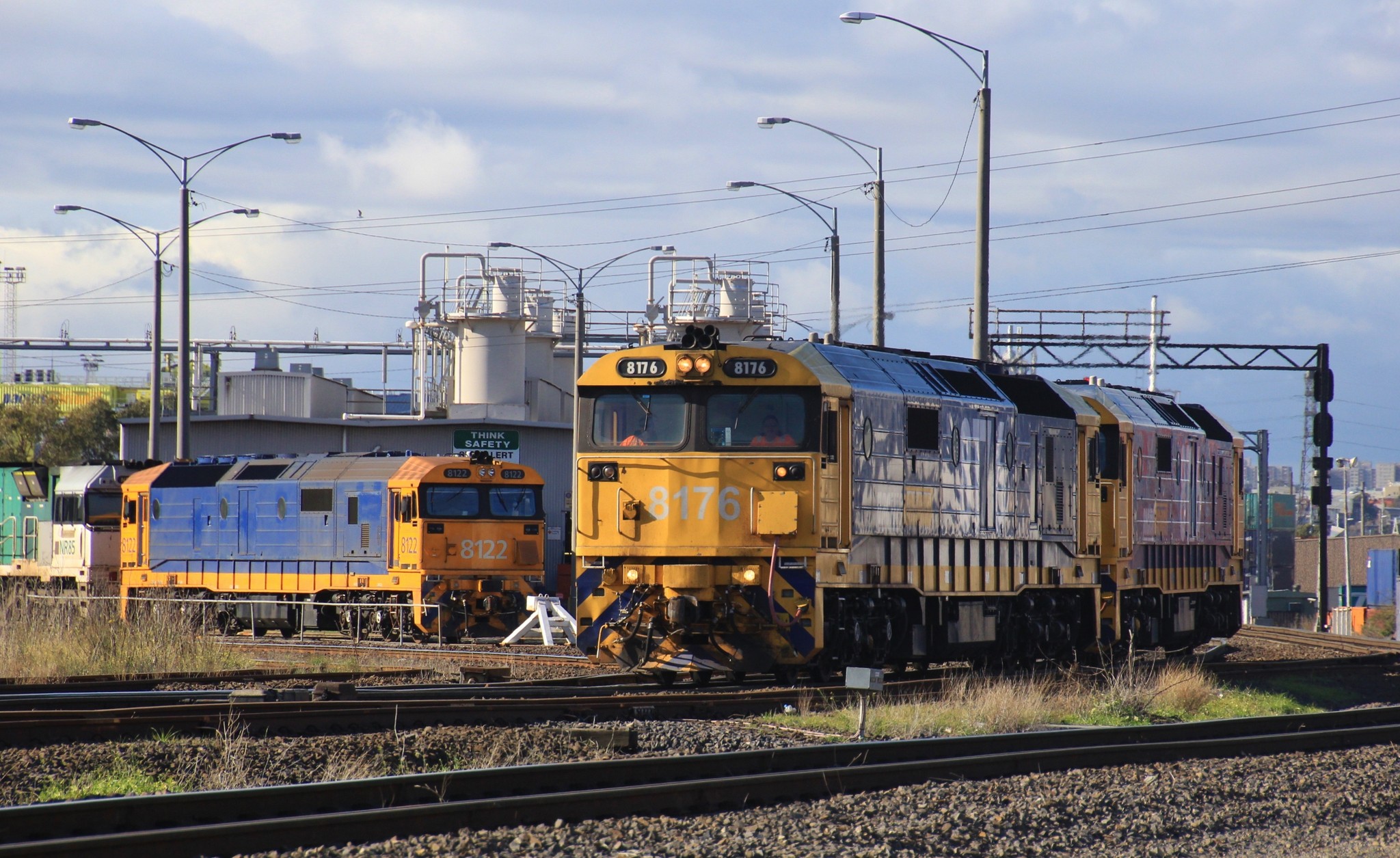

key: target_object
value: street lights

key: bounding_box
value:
[487,241,677,617]
[53,203,262,464]
[68,117,303,466]
[725,181,843,345]
[757,115,887,348]
[840,11,990,359]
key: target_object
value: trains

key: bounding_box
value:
[570,325,1245,702]
[0,444,547,650]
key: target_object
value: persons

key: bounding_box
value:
[752,417,796,448]
